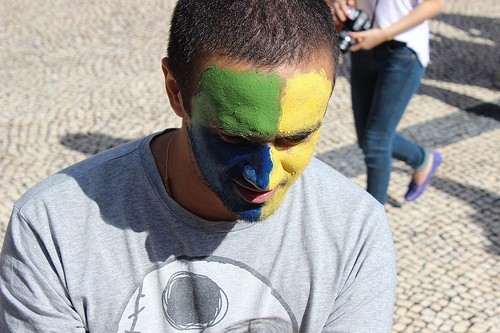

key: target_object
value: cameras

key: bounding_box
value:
[337,7,372,53]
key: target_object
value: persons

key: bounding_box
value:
[327,0,446,205]
[0,1,396,333]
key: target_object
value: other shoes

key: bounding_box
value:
[405,153,442,202]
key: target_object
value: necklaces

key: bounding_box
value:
[165,130,174,197]
